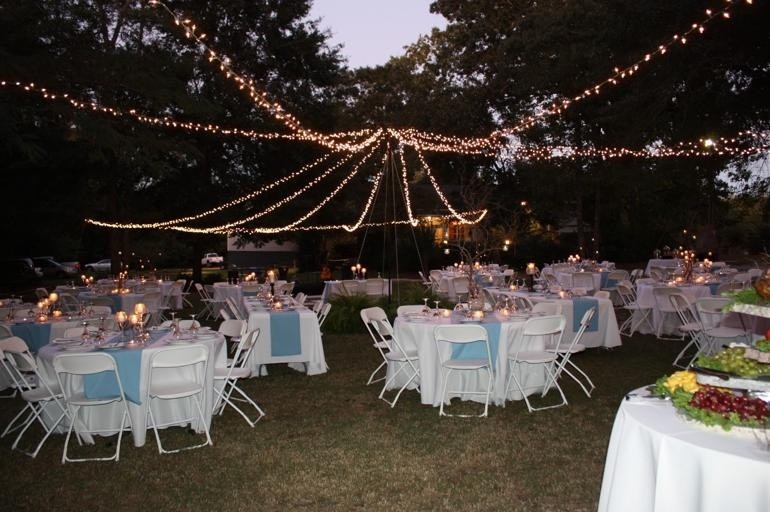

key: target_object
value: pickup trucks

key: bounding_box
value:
[201,252,224,267]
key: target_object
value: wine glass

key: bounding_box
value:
[1,271,296,350]
[422,258,746,319]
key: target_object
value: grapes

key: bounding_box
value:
[711,347,770,376]
[690,384,770,425]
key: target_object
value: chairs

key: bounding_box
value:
[359,304,556,408]
[2,350,82,458]
[433,323,497,419]
[144,344,212,455]
[196,268,332,378]
[0,257,195,399]
[54,352,135,464]
[669,293,770,374]
[541,307,596,398]
[209,328,266,428]
[525,252,762,348]
[1,303,227,446]
[418,259,516,307]
[503,315,568,412]
[322,263,393,305]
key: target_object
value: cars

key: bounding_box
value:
[0,253,123,296]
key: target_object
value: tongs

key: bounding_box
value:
[748,418,770,450]
[625,393,668,401]
[690,363,737,380]
